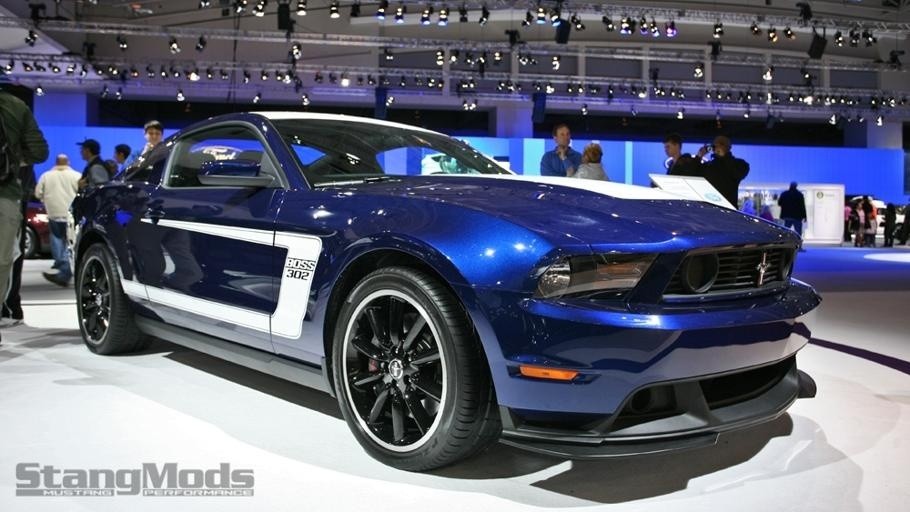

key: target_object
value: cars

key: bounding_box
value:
[24,201,51,258]
[874,207,906,235]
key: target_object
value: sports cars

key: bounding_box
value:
[66,111,823,473]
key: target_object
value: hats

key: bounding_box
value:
[76,140,100,150]
[585,144,602,162]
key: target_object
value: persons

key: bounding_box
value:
[739,190,784,226]
[572,141,610,182]
[0,88,51,347]
[843,194,910,250]
[650,132,694,189]
[687,134,750,211]
[105,158,120,178]
[778,182,808,252]
[115,143,134,179]
[42,139,110,288]
[537,122,584,176]
[138,119,166,175]
[33,154,83,269]
[0,160,37,329]
[96,186,208,296]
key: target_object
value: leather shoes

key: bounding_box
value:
[44,273,68,286]
[2,295,23,319]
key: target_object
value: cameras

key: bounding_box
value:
[705,145,716,153]
